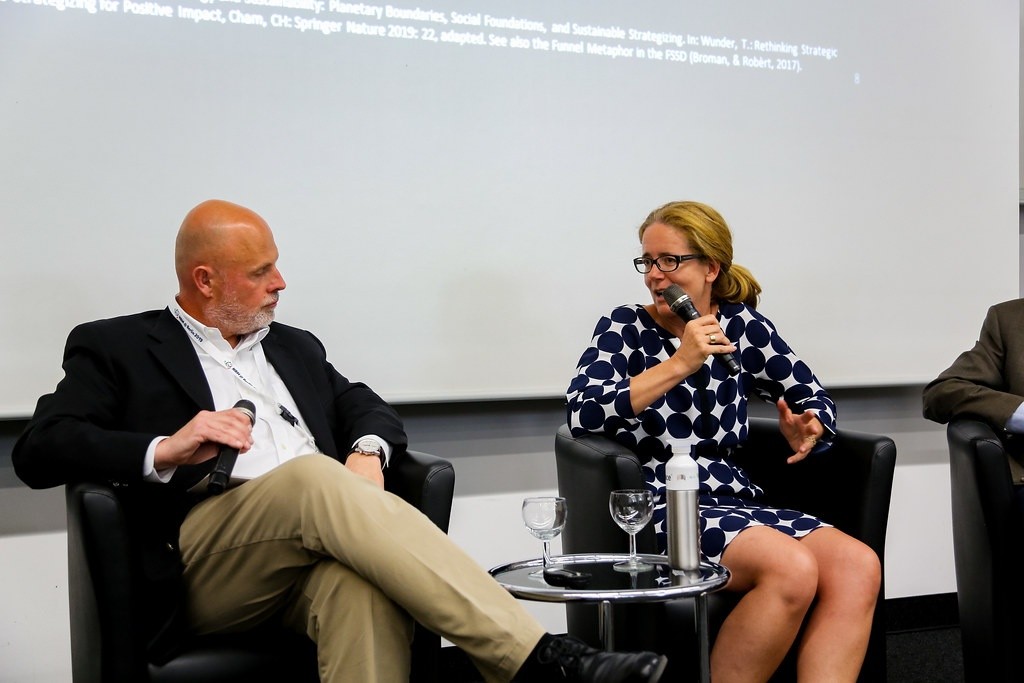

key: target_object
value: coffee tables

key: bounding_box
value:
[487,552,731,683]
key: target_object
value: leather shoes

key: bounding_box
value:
[537,636,667,683]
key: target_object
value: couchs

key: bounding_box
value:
[946,418,1024,683]
[65,441,456,683]
[556,417,897,683]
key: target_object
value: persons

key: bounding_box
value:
[563,201,882,683]
[921,298,1024,434]
[11,198,668,683]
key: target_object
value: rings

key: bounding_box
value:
[709,334,715,343]
[806,437,816,445]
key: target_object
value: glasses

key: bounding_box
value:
[634,254,704,274]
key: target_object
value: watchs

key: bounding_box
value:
[346,439,385,467]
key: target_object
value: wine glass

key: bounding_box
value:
[609,490,656,572]
[522,497,568,576]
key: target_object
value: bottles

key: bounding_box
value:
[664,439,702,569]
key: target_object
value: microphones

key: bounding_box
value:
[207,400,256,497]
[664,284,741,377]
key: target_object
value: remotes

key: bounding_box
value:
[543,568,592,589]
